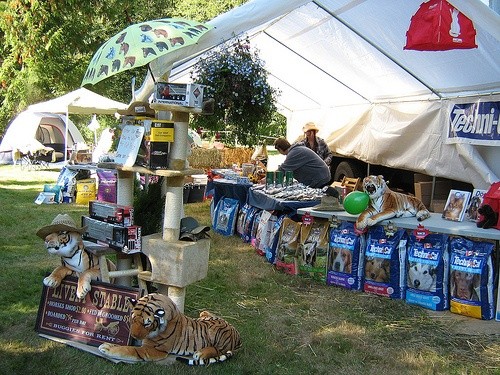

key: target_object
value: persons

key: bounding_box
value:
[260,137,332,188]
[301,122,333,166]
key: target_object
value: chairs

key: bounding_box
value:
[20,149,54,172]
[341,176,361,191]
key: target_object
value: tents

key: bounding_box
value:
[0,86,128,165]
[129,0,500,189]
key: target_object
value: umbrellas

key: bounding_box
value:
[82,11,216,85]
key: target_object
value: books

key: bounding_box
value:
[441,189,490,222]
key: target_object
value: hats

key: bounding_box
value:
[180,216,211,235]
[180,231,205,242]
[303,122,319,134]
[36,214,89,241]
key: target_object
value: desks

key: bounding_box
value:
[74,153,92,165]
[246,187,321,222]
[82,240,149,296]
[212,180,253,205]
[297,207,500,322]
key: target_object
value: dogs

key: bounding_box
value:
[448,270,481,302]
[441,192,465,222]
[301,243,317,266]
[466,196,481,222]
[364,256,390,283]
[406,261,437,292]
[328,246,352,274]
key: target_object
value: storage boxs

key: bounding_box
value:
[414,181,449,208]
[81,200,142,254]
[183,183,206,204]
[122,119,174,169]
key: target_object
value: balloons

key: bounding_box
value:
[343,191,370,215]
